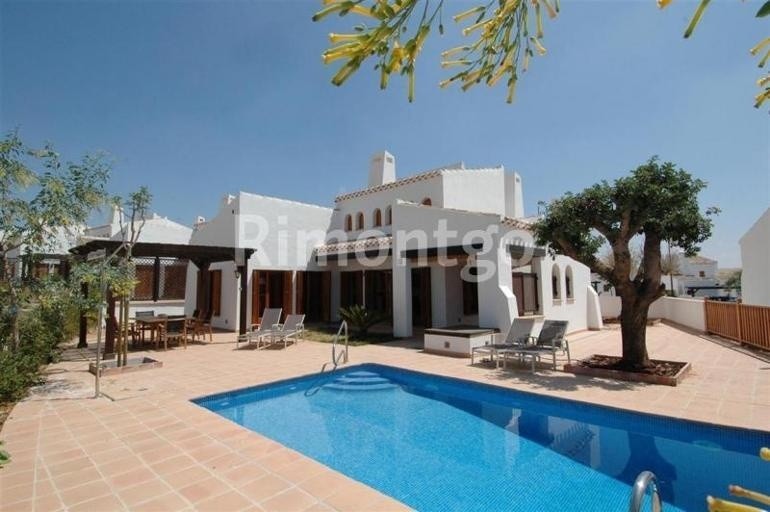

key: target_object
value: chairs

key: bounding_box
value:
[116,309,216,351]
[471,317,535,370]
[236,307,283,349]
[504,319,570,375]
[257,314,306,351]
